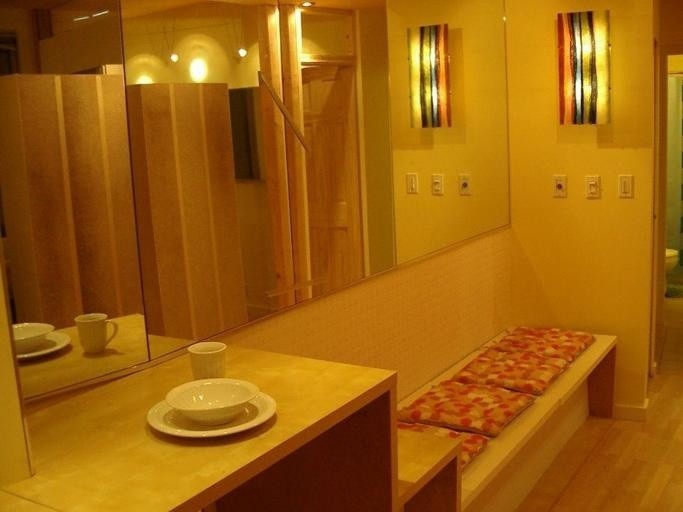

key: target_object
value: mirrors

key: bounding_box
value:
[0,0,512,406]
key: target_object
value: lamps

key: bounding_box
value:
[407,24,451,129]
[557,9,610,125]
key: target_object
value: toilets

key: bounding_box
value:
[663,249,678,296]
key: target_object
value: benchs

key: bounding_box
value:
[398,328,617,512]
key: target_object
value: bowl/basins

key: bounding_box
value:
[10,323,57,352]
[164,377,258,424]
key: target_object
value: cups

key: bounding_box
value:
[73,312,118,355]
[188,341,226,375]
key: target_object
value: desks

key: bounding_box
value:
[396,427,464,512]
[17,313,197,411]
[0,344,398,512]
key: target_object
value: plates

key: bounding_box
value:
[146,394,277,438]
[13,334,69,360]
